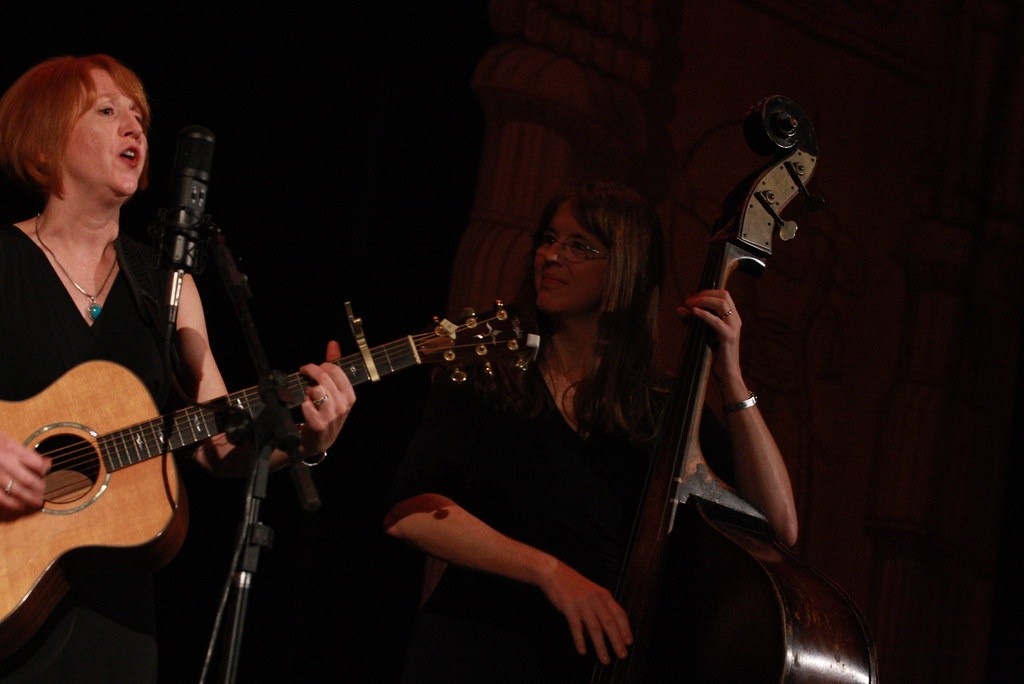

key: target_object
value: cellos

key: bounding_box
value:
[578,93,882,684]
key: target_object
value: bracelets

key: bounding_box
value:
[721,391,758,411]
[295,423,327,467]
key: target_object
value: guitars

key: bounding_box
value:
[0,296,543,662]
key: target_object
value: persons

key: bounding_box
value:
[0,54,357,684]
[373,178,798,684]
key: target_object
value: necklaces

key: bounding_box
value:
[36,213,117,321]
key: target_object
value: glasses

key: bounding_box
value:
[533,231,610,263]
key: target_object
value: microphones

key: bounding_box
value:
[162,126,216,325]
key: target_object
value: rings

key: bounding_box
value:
[314,394,330,406]
[4,480,14,495]
[722,310,732,316]
